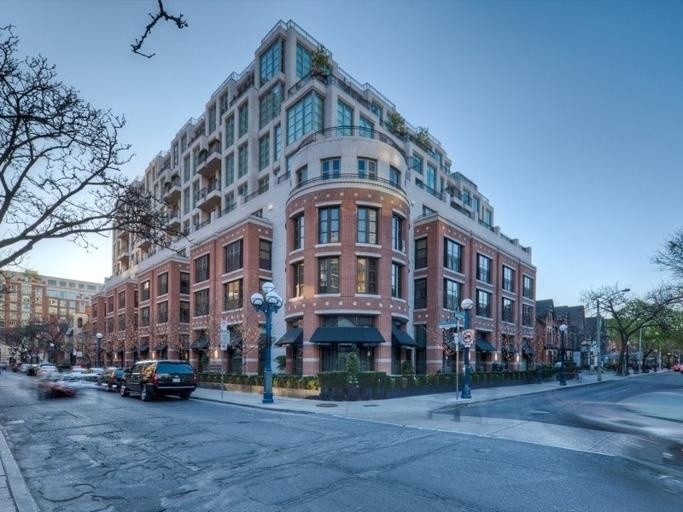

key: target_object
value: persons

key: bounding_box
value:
[674,361,683,377]
[554,360,566,369]
[478,357,486,371]
[607,358,620,371]
[492,360,504,371]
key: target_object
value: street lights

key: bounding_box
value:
[558,324,569,386]
[460,299,475,401]
[95,332,103,367]
[595,287,632,380]
[49,342,54,364]
[250,282,282,404]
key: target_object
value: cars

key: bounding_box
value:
[11,362,61,378]
[0,362,8,370]
[33,378,75,397]
[62,368,123,391]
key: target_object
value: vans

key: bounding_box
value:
[120,360,197,401]
